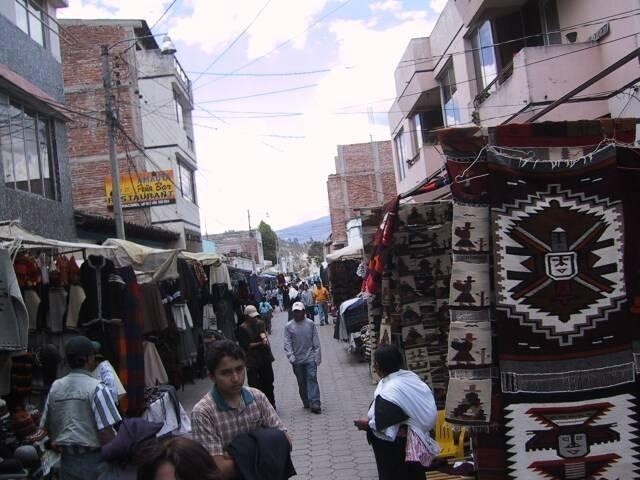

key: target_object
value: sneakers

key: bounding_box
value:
[311,404,321,411]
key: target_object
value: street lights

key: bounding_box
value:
[100,32,178,241]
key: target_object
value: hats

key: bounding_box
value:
[461,333,477,342]
[464,385,481,392]
[244,305,260,319]
[292,302,306,311]
[464,276,475,282]
[66,335,101,358]
[39,344,62,365]
[462,222,474,229]
[0,397,47,479]
